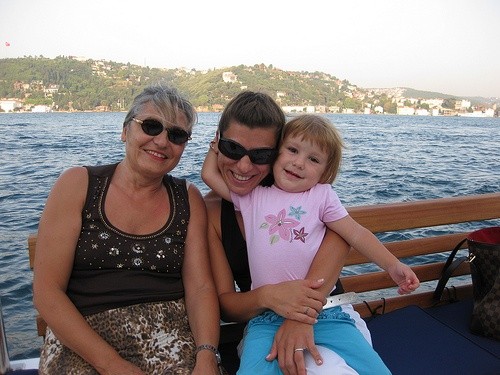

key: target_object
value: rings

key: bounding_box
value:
[295,349,303,351]
[303,307,309,313]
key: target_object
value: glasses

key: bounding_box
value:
[132,118,192,145]
[218,130,277,165]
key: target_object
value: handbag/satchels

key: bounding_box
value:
[432,226,500,340]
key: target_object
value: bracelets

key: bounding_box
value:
[197,345,221,364]
[209,143,219,154]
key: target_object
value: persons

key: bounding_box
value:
[33,86,224,375]
[202,113,419,375]
[204,92,371,375]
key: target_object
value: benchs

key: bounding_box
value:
[27,192,500,375]
